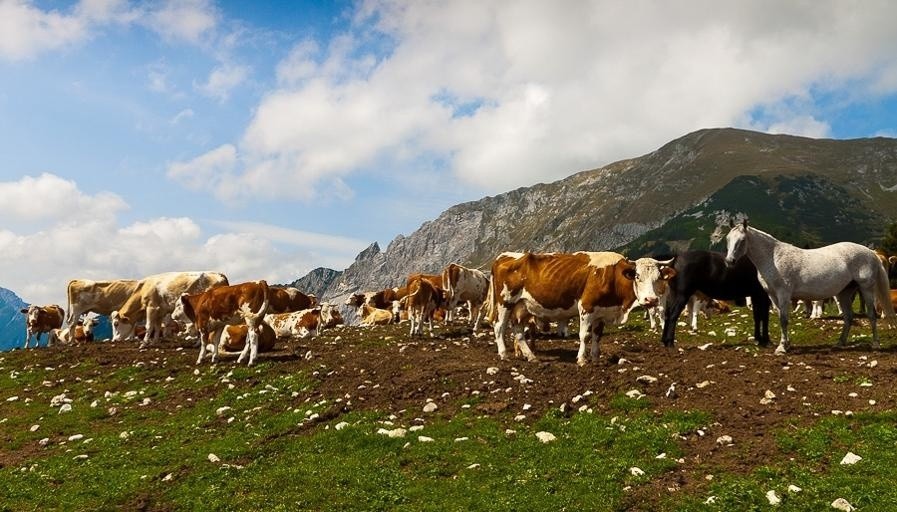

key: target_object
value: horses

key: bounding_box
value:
[661,217,896,357]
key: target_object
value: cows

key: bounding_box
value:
[19,303,65,350]
[343,251,897,368]
[63,270,342,368]
[45,314,100,349]
